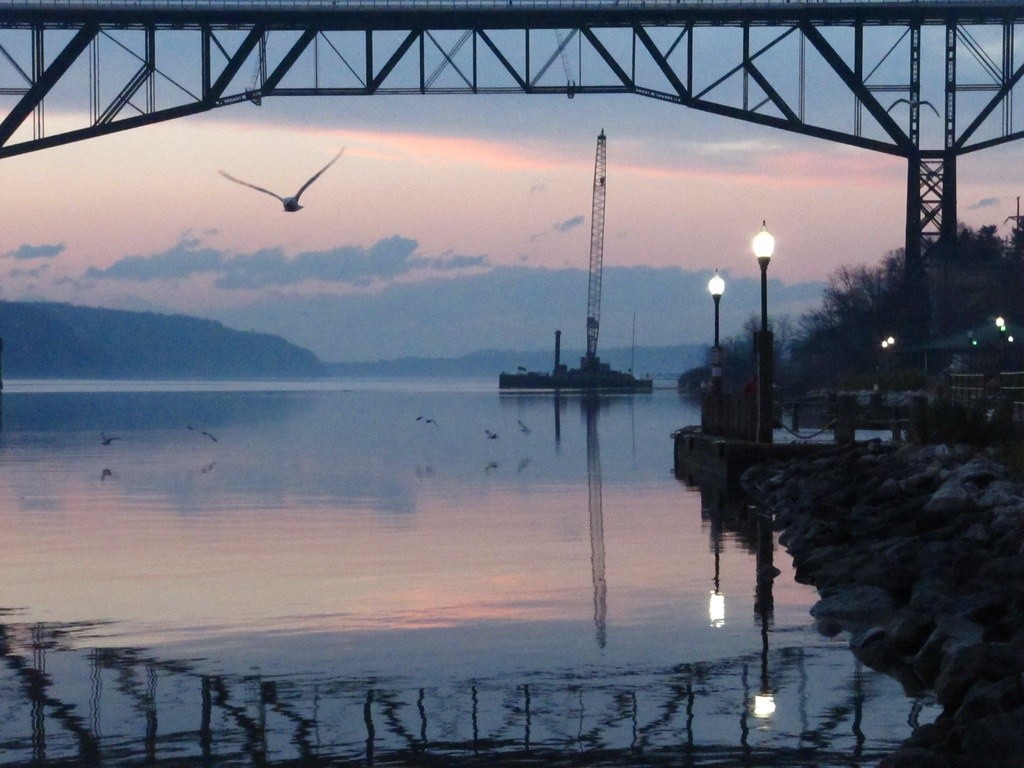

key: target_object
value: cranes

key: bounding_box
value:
[580,127,607,364]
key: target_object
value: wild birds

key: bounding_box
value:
[483,418,532,440]
[186,422,219,443]
[415,416,440,429]
[215,146,347,213]
[100,431,122,446]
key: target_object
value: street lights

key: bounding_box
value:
[752,218,775,444]
[706,266,727,432]
